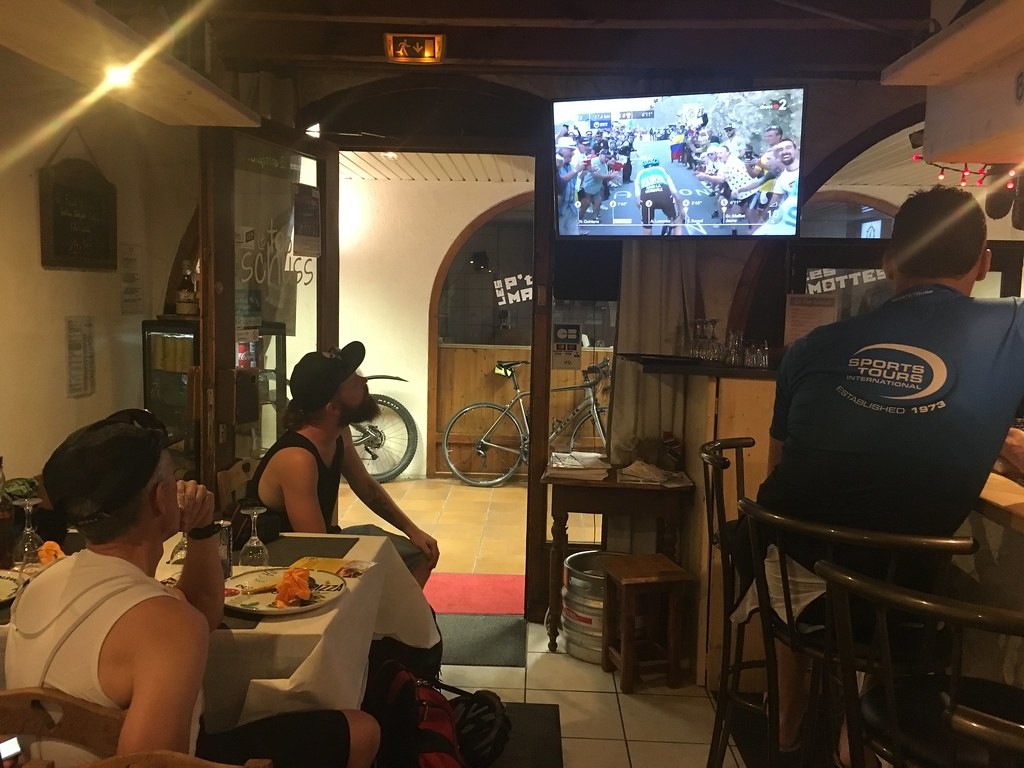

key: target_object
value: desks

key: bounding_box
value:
[540,462,695,652]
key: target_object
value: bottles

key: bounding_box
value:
[176,260,198,315]
[0,456,15,570]
[238,336,267,370]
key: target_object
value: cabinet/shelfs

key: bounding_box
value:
[141,316,287,510]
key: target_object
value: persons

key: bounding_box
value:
[5,410,380,768]
[245,341,440,591]
[556,120,800,235]
[751,184,1024,768]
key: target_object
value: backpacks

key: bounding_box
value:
[13,507,67,545]
[378,668,470,768]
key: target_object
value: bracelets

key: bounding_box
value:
[188,521,222,540]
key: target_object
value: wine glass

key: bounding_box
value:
[11,498,44,574]
[239,506,269,566]
[678,319,769,369]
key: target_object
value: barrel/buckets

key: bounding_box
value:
[561,550,660,666]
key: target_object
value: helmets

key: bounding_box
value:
[450,689,511,768]
[641,154,658,167]
[5,478,38,496]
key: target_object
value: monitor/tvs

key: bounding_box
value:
[551,84,809,242]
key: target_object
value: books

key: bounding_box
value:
[544,451,612,481]
[617,461,694,489]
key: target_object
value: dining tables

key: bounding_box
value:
[0,528,441,734]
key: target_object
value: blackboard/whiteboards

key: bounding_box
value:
[39,158,119,272]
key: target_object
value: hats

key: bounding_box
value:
[43,418,187,527]
[555,137,577,149]
[579,137,590,146]
[290,341,365,411]
[597,149,611,154]
[724,123,736,130]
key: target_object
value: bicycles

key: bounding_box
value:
[440,356,613,488]
[259,367,418,483]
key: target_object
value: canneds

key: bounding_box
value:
[237,341,258,368]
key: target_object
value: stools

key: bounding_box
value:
[601,554,692,694]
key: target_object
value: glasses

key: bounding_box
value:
[765,126,779,130]
[706,152,716,157]
[587,134,592,135]
[726,129,732,132]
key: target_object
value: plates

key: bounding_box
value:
[224,567,347,614]
[0,564,42,603]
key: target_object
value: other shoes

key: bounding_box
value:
[779,737,805,768]
[622,180,633,184]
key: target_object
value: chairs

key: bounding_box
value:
[736,498,977,768]
[816,559,1024,768]
[698,437,780,768]
